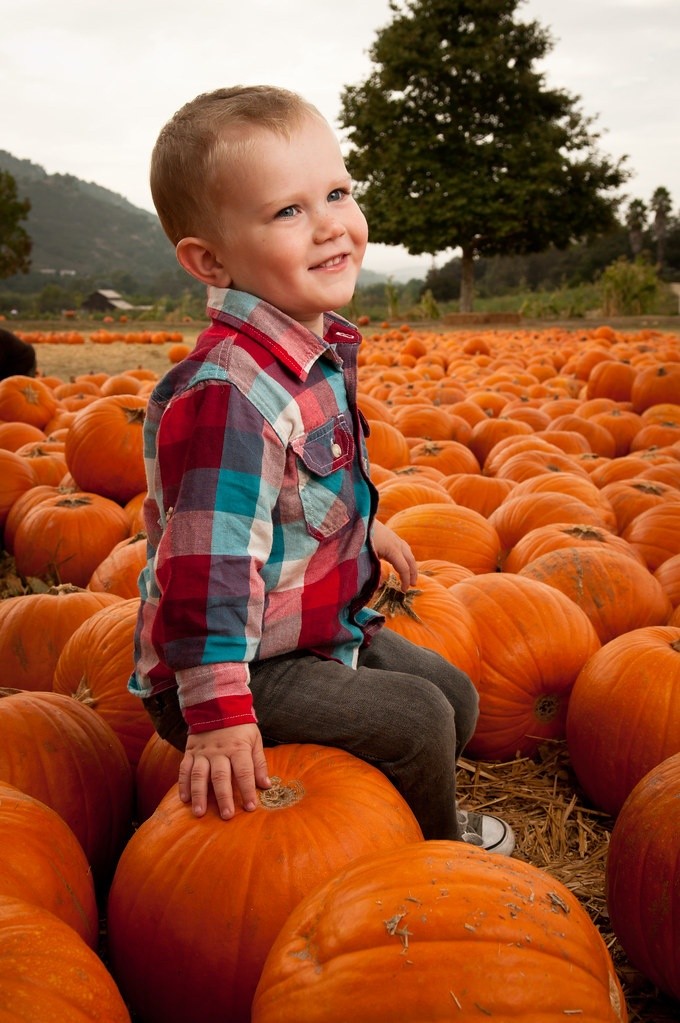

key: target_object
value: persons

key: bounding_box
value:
[125,86,515,853]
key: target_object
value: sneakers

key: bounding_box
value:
[454,809,515,856]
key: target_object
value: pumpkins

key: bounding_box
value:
[0,314,680,1023]
